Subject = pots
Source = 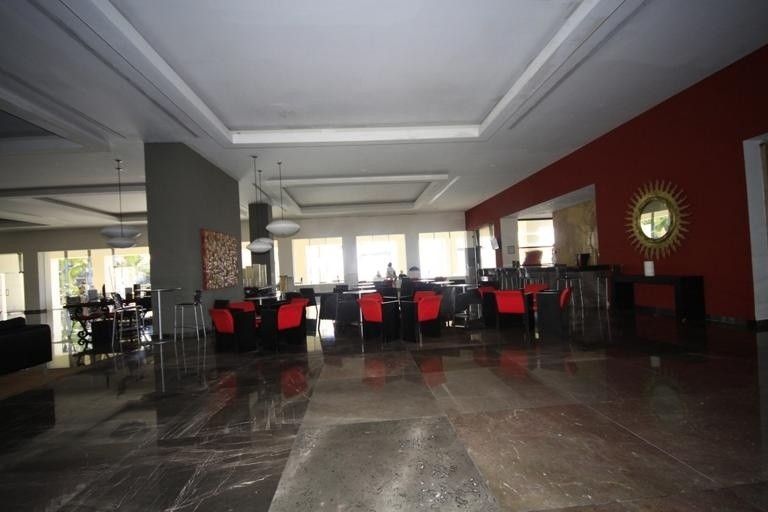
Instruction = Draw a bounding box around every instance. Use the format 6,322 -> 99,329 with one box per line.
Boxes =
576,252 -> 590,267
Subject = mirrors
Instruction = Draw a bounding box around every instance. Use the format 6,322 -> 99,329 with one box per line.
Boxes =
622,179 -> 693,259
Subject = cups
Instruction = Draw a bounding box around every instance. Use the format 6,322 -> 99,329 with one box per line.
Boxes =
88,288 -> 97,301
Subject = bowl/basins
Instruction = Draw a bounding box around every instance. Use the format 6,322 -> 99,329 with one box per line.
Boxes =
67,296 -> 83,306
242,263 -> 267,288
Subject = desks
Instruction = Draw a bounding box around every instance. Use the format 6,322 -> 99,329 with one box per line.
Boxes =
135,287 -> 183,344
612,274 -> 707,340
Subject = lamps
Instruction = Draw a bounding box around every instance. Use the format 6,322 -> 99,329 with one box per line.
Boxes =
254,170 -> 275,244
105,159 -> 136,248
266,161 -> 300,238
99,168 -> 143,240
246,155 -> 272,254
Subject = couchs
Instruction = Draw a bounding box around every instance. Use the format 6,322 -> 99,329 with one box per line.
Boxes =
0,388 -> 55,456
0,316 -> 52,377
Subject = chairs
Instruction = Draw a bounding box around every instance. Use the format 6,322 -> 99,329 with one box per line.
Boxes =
472,331 -> 577,386
319,337 -> 462,391
174,290 -> 206,343
64,284 -> 149,358
204,361 -> 311,435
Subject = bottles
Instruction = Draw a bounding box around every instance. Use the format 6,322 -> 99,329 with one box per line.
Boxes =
336,274 -> 340,283
300,276 -> 303,284
125,284 -> 140,298
511,257 -> 520,268
